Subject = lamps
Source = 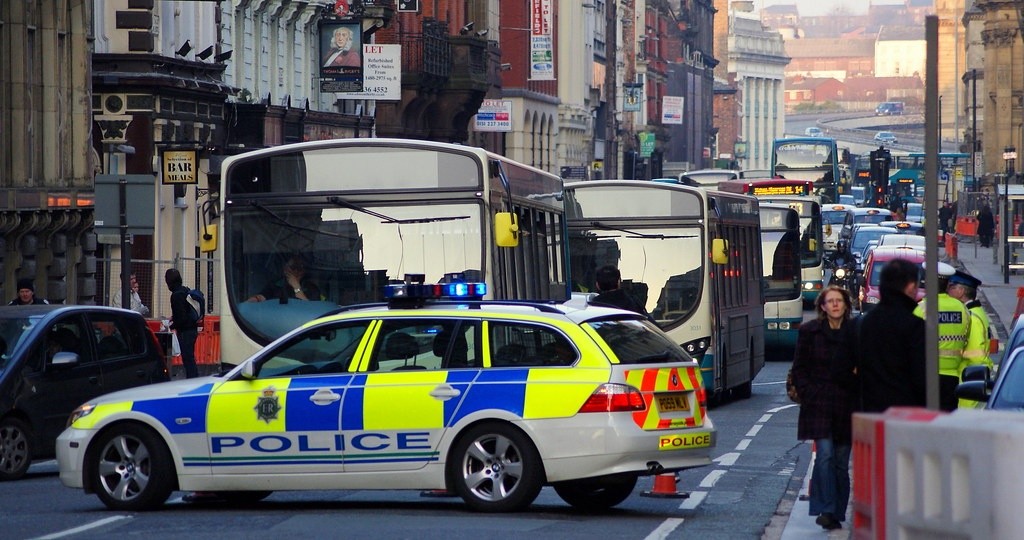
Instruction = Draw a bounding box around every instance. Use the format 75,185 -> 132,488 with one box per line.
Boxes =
214,50 -> 233,62
109,144 -> 135,154
175,40 -> 191,57
475,29 -> 489,38
195,45 -> 213,60
461,22 -> 475,35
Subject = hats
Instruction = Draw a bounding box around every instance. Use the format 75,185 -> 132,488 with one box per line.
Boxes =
920,260 -> 957,283
17,278 -> 34,292
948,270 -> 982,288
165,269 -> 180,285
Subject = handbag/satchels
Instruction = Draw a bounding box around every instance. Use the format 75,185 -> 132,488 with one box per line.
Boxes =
168,327 -> 181,357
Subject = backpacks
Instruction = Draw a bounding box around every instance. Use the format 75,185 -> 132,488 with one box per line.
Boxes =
172,287 -> 205,325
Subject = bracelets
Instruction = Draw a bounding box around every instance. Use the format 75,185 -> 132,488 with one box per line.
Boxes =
294,288 -> 302,294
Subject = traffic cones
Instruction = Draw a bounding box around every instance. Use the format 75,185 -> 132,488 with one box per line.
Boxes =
640,473 -> 690,499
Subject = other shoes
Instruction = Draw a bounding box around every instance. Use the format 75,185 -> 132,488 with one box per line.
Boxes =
816,513 -> 841,529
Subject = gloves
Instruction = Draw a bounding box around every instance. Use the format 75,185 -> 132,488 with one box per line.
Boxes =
132,303 -> 149,314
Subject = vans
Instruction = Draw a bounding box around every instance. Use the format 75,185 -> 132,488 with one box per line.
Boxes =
875,102 -> 904,117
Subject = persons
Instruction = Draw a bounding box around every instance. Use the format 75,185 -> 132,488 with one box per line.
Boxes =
841,258 -> 929,412
976,205 -> 994,248
592,263 -> 640,314
792,287 -> 860,529
7,279 -> 48,305
165,268 -> 199,379
891,190 -> 908,221
946,270 -> 994,413
244,256 -> 322,302
112,271 -> 149,318
910,260 -> 971,414
939,200 -> 956,243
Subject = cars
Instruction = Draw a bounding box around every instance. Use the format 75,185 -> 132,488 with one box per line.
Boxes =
0,304 -> 173,484
54,275 -> 717,513
956,314 -> 1024,412
875,131 -> 899,144
805,127 -> 823,138
819,185 -> 940,314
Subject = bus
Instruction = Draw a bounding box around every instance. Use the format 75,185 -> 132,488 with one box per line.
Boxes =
561,177 -> 765,412
837,147 -> 979,207
198,137 -> 571,378
651,167 -> 833,356
768,136 -> 840,205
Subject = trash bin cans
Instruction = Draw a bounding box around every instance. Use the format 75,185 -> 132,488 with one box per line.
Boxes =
170,314 -> 220,374
992,238 -> 1000,264
956,216 -> 979,236
154,332 -> 174,371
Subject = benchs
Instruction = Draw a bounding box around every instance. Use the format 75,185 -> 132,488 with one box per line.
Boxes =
496,342 -> 572,365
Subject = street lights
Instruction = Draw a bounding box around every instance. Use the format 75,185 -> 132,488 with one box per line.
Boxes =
1002,145 -> 1016,284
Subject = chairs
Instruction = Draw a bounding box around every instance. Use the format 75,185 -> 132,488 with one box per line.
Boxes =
384,328 -> 469,370
44,326 -> 83,363
310,276 -> 331,302
